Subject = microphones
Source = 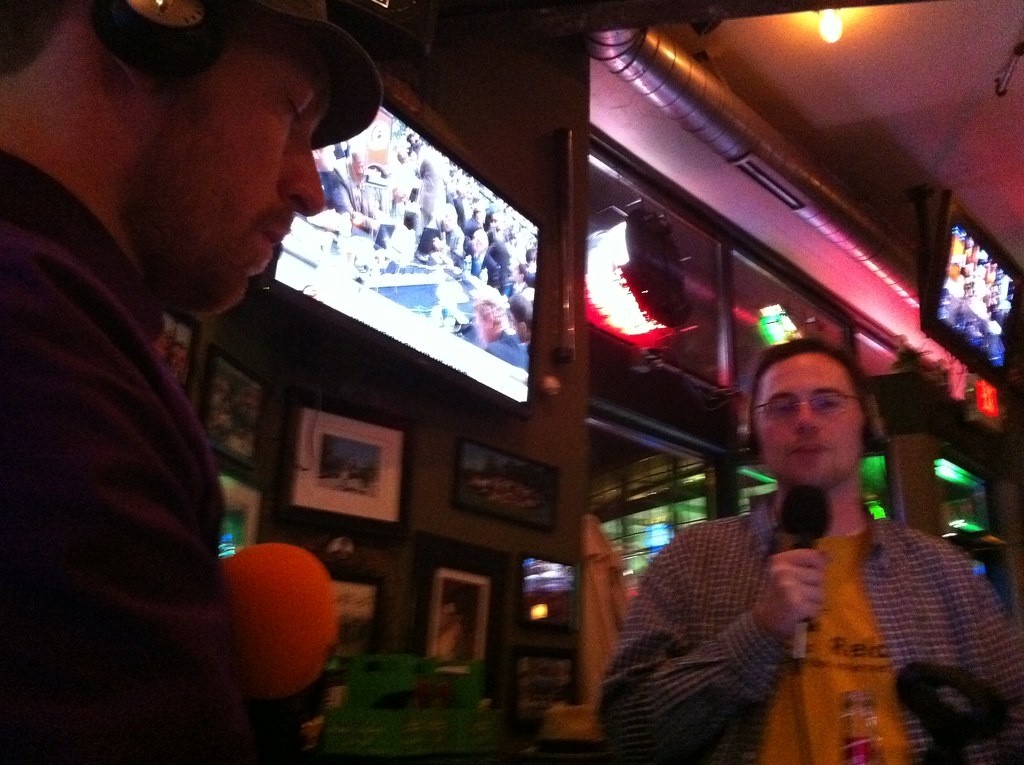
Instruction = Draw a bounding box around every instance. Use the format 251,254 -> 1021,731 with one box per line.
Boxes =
224,543 -> 338,765
782,484 -> 832,661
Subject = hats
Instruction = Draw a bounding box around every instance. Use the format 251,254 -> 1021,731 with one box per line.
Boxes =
206,0 -> 383,147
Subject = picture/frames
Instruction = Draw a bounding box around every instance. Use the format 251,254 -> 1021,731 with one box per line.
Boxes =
152,302 -> 205,399
450,437 -> 561,531
508,643 -> 578,731
269,387 -> 416,541
515,552 -> 581,632
403,530 -> 510,699
199,344 -> 273,470
216,462 -> 269,560
323,562 -> 385,670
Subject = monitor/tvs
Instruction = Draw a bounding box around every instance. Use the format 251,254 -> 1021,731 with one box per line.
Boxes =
921,186 -> 1024,387
255,93 -> 548,420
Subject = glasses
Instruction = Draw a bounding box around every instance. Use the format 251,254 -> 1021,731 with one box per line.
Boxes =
752,392 -> 863,422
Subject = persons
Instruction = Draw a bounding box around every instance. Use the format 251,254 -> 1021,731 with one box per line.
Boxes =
961,233 -> 1006,322
595,338 -> 1024,765
312,122 -> 538,368
0,0 -> 378,765
